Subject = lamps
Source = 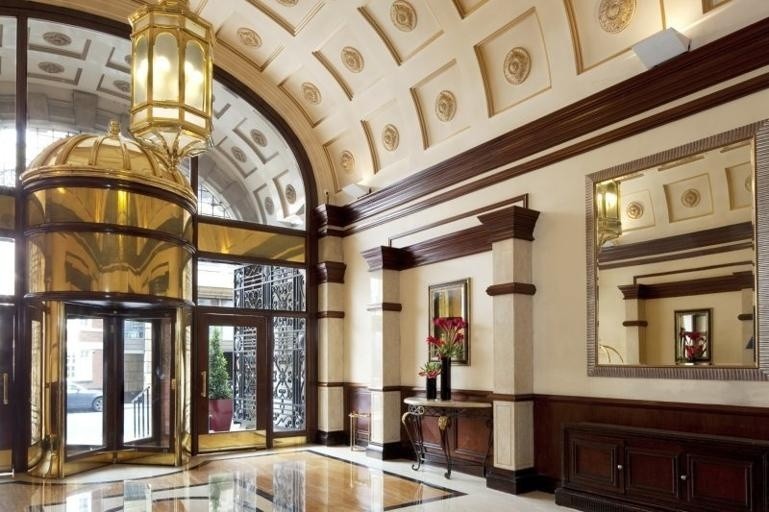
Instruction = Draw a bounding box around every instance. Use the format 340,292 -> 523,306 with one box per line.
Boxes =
595,178 -> 623,256
126,2 -> 219,175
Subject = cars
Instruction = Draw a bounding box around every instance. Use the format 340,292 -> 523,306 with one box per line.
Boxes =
66,380 -> 103,413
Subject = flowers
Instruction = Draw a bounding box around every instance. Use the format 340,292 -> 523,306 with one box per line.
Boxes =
679,325 -> 707,346
418,361 -> 442,378
426,315 -> 469,361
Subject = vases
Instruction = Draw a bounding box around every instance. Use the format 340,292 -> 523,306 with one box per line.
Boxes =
440,355 -> 452,400
685,346 -> 702,362
426,376 -> 436,400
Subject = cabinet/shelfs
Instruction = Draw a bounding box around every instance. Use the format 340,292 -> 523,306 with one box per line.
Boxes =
555,419 -> 769,511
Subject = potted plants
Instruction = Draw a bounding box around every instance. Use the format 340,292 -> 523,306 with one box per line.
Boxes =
208,325 -> 236,431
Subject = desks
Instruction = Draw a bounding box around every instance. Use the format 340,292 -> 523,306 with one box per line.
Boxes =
400,395 -> 493,479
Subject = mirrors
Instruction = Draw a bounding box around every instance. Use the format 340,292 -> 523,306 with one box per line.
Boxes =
673,307 -> 713,364
584,117 -> 768,384
427,275 -> 474,367
